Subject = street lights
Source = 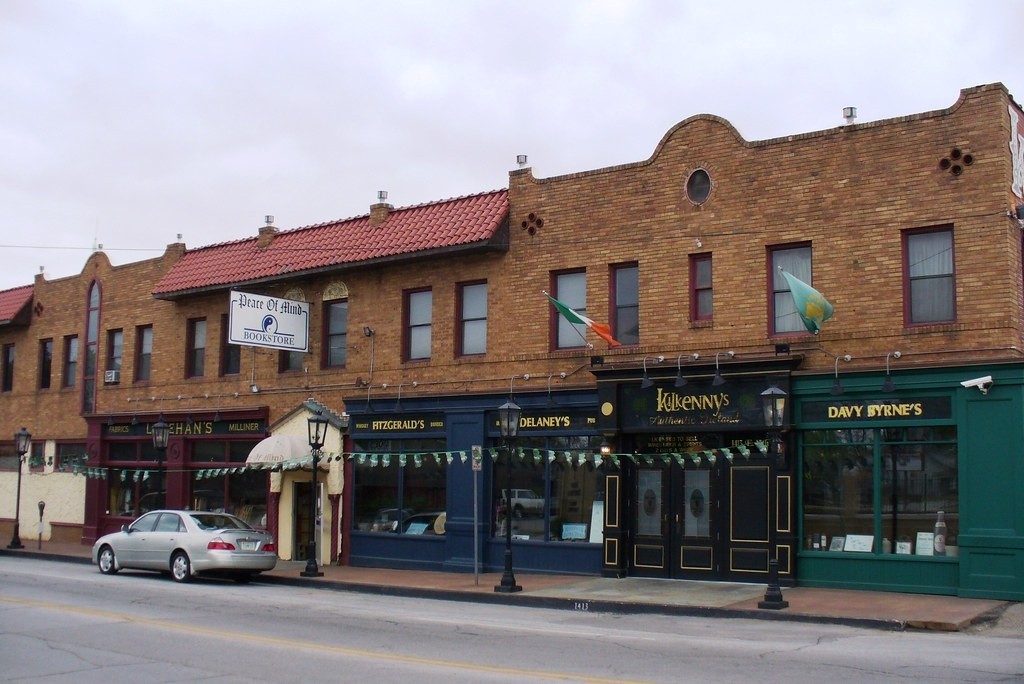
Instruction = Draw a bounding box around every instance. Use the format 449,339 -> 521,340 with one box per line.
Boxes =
6,427 -> 31,549
758,385 -> 789,610
493,398 -> 523,593
300,411 -> 329,577
882,428 -> 904,553
151,412 -> 171,508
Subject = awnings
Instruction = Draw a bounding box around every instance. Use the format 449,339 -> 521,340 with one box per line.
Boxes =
246,434 -> 329,472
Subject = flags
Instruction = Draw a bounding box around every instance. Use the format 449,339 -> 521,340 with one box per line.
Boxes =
782,271 -> 833,332
547,294 -> 621,346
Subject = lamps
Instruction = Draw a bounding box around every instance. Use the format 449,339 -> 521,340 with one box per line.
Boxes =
674,353 -> 700,388
364,384 -> 387,414
642,356 -> 663,388
712,352 -> 733,387
544,372 -> 566,409
394,381 -> 418,412
106,397 -> 226,424
830,355 -> 851,394
507,373 -> 530,404
881,351 -> 902,393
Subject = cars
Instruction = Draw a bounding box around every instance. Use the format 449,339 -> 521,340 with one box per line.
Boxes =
373,509 -> 414,530
91,509 -> 278,583
402,512 -> 446,535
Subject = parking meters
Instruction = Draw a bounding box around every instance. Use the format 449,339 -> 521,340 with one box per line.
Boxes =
37,500 -> 45,549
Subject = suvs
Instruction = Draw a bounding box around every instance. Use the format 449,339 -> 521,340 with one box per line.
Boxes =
500,489 -> 544,519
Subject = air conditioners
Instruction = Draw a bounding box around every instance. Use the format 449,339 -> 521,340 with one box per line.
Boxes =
105,370 -> 119,383
